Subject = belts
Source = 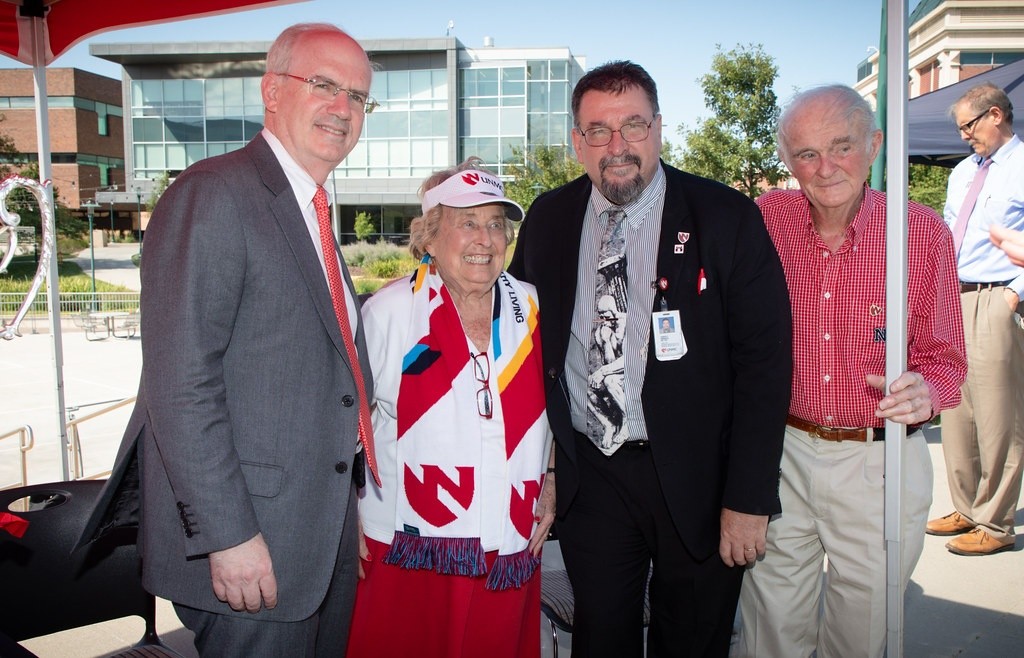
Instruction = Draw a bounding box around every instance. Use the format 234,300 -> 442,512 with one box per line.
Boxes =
957,282 -> 1012,293
788,416 -> 921,443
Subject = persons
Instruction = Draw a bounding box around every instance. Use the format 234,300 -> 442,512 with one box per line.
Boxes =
947,81 -> 1024,556
989,224 -> 1024,268
360,156 -> 557,658
70,22 -> 382,658
506,60 -> 792,658
736,85 -> 966,658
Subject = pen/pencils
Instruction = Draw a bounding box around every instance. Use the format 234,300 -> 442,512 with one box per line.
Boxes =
697,268 -> 707,295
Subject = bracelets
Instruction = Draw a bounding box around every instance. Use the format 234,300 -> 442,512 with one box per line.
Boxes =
547,468 -> 555,473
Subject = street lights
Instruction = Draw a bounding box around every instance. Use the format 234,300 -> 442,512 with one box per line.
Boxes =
110,198 -> 115,243
80,197 -> 102,311
132,186 -> 145,255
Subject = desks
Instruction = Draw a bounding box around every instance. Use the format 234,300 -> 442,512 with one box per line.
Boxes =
88,312 -> 129,338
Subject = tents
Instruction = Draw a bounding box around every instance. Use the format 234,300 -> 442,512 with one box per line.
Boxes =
0,0 -> 309,483
907,57 -> 1024,167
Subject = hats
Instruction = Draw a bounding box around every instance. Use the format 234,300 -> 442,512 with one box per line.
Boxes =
422,169 -> 525,224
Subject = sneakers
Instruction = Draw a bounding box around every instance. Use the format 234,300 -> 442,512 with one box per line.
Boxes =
926,510 -> 978,536
944,528 -> 1015,556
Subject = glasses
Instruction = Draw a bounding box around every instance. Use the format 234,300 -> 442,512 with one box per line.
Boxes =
955,108 -> 990,136
273,71 -> 380,117
575,118 -> 659,146
470,349 -> 498,420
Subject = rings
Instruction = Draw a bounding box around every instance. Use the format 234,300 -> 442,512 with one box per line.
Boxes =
744,547 -> 756,551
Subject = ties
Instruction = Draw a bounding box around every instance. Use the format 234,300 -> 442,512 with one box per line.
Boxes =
586,208 -> 633,457
311,183 -> 384,491
951,159 -> 994,266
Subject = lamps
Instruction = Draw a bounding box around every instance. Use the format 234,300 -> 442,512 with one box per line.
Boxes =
867,47 -> 878,51
446,20 -> 454,36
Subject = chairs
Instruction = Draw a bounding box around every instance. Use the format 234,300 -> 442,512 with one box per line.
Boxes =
537,520 -> 653,658
0,480 -> 185,658
71,308 -> 140,341
